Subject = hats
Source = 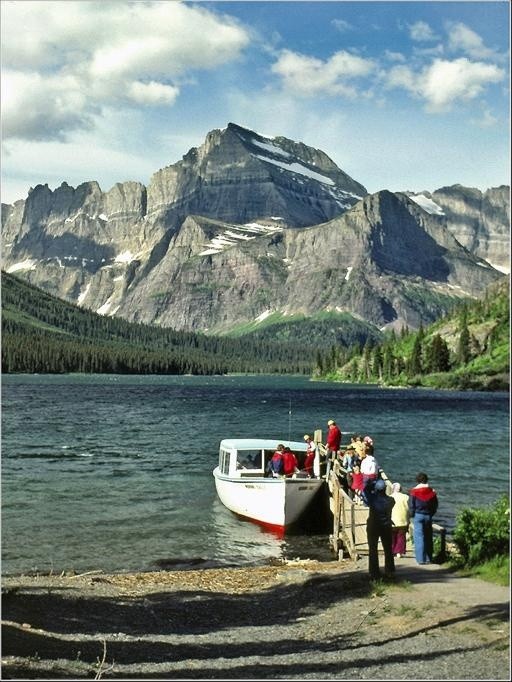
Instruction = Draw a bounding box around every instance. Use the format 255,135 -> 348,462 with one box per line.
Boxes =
346,445 -> 355,450
303,435 -> 310,440
328,420 -> 334,426
375,480 -> 386,491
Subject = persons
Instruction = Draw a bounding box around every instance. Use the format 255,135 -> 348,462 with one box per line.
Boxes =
269,420 -> 410,579
408,471 -> 438,565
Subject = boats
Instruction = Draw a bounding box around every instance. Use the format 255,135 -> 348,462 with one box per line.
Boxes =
212,438 -> 325,541
